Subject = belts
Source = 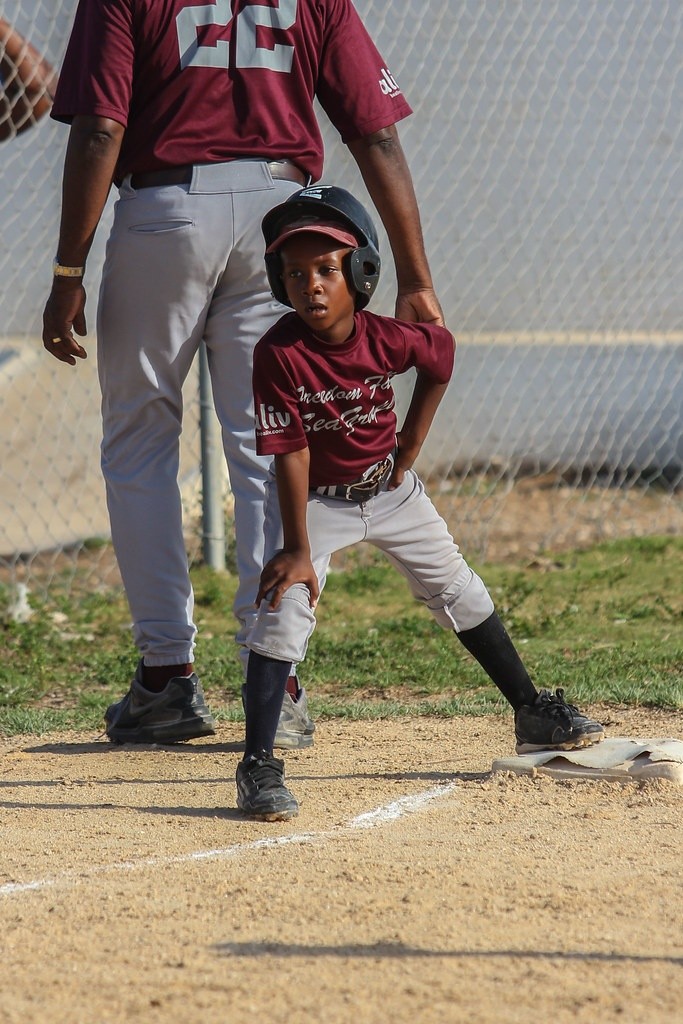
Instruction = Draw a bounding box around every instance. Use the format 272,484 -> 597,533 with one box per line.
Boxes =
310,459 -> 394,501
130,159 -> 309,189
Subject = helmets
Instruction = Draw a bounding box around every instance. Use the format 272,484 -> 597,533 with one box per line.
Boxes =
262,185 -> 381,310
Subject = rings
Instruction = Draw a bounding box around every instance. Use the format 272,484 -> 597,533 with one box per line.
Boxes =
52,336 -> 62,344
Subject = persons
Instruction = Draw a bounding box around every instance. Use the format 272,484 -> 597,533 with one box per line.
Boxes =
0,11 -> 58,152
236,184 -> 601,823
40,0 -> 445,746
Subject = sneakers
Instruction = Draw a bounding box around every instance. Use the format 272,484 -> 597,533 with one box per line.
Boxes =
236,749 -> 299,821
241,675 -> 316,749
98,655 -> 215,744
514,689 -> 605,754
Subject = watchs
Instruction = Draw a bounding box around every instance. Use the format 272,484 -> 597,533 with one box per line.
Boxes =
47,254 -> 88,280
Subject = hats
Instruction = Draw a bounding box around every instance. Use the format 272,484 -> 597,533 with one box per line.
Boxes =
266,209 -> 360,253
